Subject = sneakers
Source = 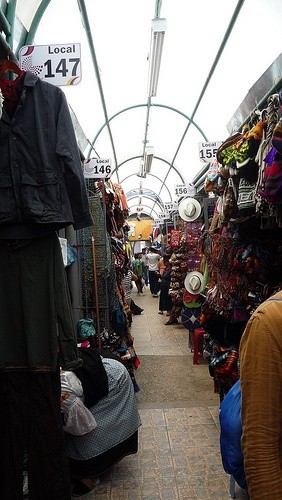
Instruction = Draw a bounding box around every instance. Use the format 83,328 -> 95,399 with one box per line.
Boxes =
166,313 -> 170,316
158,311 -> 163,314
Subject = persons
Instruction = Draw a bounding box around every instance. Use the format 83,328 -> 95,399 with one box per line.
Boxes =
240,291 -> 282,500
121,247 -> 178,325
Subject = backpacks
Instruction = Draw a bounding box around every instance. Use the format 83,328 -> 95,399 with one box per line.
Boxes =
219,378 -> 247,489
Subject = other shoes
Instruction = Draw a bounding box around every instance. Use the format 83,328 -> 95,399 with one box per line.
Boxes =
153,294 -> 158,297
134,308 -> 144,315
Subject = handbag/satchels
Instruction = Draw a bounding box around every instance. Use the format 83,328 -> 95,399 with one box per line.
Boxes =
78,318 -> 96,338
204,90 -> 282,222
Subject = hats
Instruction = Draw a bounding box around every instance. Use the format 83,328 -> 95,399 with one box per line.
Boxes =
181,308 -> 201,331
185,271 -> 205,294
179,198 -> 202,221
183,290 -> 202,307
